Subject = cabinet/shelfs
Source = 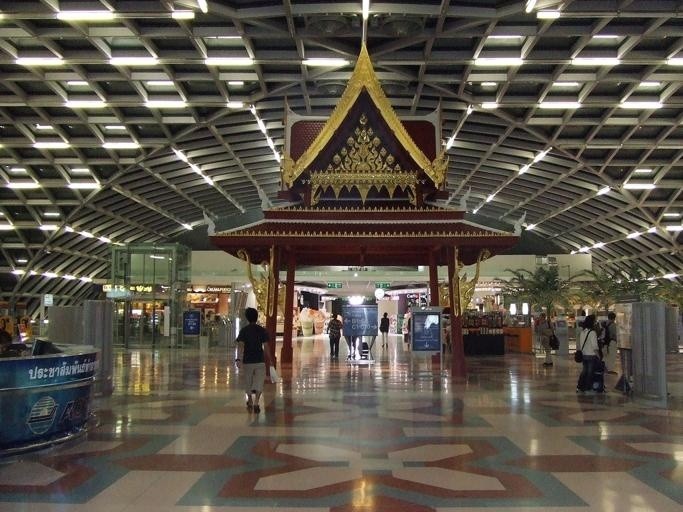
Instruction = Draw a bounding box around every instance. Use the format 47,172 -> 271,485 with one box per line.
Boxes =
406,289 -> 431,308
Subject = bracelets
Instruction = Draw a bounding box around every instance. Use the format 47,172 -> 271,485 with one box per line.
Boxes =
236,359 -> 240,362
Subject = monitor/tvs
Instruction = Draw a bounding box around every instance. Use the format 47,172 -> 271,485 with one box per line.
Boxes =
31,337 -> 51,355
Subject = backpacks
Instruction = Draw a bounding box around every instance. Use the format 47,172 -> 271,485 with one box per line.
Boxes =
597,321 -> 616,354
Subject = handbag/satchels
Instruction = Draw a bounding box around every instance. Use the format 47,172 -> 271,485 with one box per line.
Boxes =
575,350 -> 583,362
269,366 -> 280,384
550,335 -> 560,350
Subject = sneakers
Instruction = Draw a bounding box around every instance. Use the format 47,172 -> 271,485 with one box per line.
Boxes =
608,371 -> 617,374
246,401 -> 253,408
544,362 -> 553,367
382,344 -> 388,348
254,408 -> 260,413
615,385 -> 633,392
576,388 -> 597,394
331,356 -> 338,359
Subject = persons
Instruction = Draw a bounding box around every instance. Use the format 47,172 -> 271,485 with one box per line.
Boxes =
407,318 -> 411,348
22,319 -> 28,328
379,312 -> 389,348
576,312 -> 618,395
0,319 -> 6,332
236,307 -> 274,414
327,313 -> 343,357
0,334 -> 9,357
537,313 -> 554,367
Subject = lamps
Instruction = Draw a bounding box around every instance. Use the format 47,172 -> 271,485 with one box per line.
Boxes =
374,288 -> 391,300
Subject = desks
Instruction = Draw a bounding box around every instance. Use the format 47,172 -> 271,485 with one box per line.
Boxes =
503,327 -> 532,353
463,335 -> 505,355
